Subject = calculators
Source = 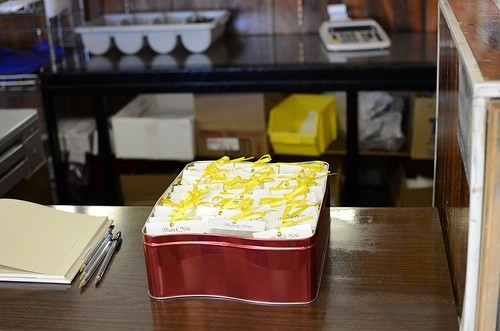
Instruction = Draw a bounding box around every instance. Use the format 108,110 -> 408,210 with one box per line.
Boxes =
321,17 -> 391,52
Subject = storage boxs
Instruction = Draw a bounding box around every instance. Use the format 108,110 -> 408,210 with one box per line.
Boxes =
119,172 -> 177,206
198,93 -> 279,157
141,161 -> 330,305
268,94 -> 337,156
408,93 -> 436,159
388,164 -> 433,207
111,92 -> 197,161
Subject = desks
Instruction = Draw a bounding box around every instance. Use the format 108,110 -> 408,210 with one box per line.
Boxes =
0,205 -> 460,331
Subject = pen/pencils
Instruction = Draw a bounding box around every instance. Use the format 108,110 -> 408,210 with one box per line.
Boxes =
93,231 -> 121,284
79,225 -> 115,271
79,239 -> 112,288
80,232 -> 112,282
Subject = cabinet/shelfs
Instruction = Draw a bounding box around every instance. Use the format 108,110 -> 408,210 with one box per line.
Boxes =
0,0 -> 90,205
38,31 -> 439,208
